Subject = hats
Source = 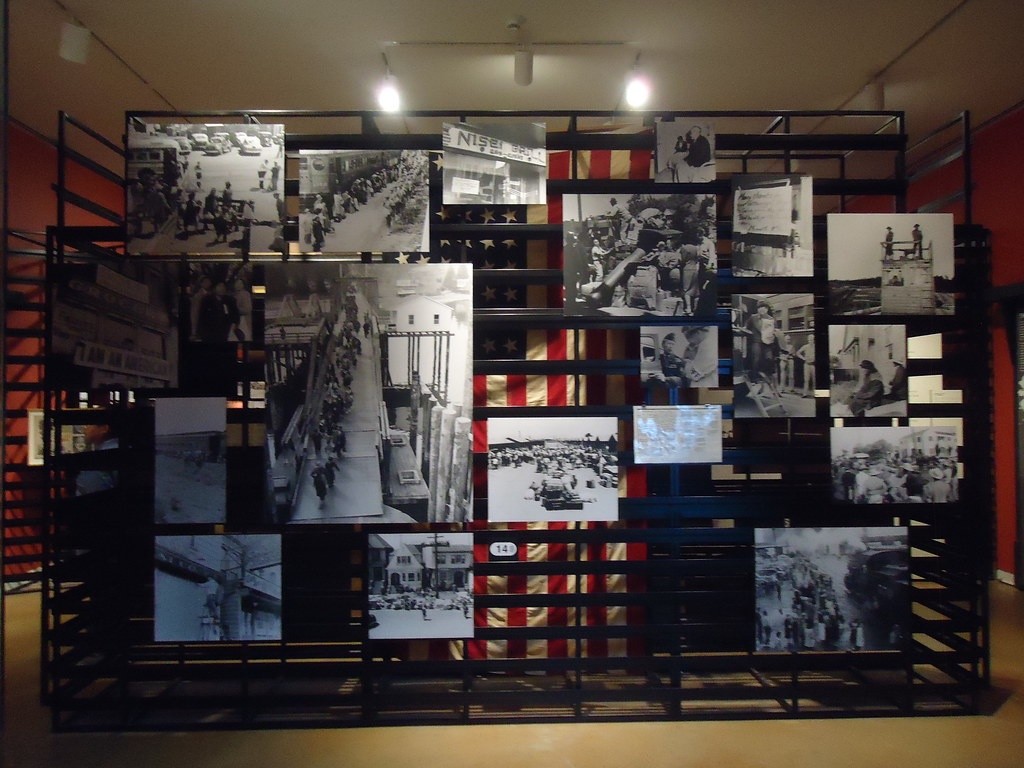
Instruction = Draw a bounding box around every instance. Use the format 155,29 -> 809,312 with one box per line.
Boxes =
859,465 -> 868,469
899,463 -> 914,472
900,475 -> 929,488
913,224 -> 920,227
859,359 -> 878,372
929,468 -> 943,479
759,300 -> 771,308
886,227 -> 892,229
609,198 -> 617,203
867,468 -> 882,475
888,355 -> 904,365
316,194 -> 322,199
912,465 -> 921,472
662,332 -> 676,344
205,273 -> 227,291
944,463 -> 957,475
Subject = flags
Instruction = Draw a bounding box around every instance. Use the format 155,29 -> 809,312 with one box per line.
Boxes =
382,146 -> 655,677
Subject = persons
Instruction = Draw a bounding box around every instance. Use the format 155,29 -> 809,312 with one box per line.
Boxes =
190,274 -> 253,343
68,402 -> 121,556
660,326 -> 718,388
303,154 -> 429,253
197,576 -> 220,619
847,358 -> 907,417
674,125 -> 710,182
488,445 -> 607,496
308,286 -> 371,501
744,303 -> 816,398
831,448 -> 959,503
756,573 -> 863,650
181,445 -> 205,473
378,582 -> 473,620
882,224 -> 925,260
564,196 -> 717,316
139,159 -> 284,243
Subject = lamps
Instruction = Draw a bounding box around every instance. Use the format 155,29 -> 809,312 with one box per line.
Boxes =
514,49 -> 534,87
865,83 -> 885,111
56,21 -> 91,67
